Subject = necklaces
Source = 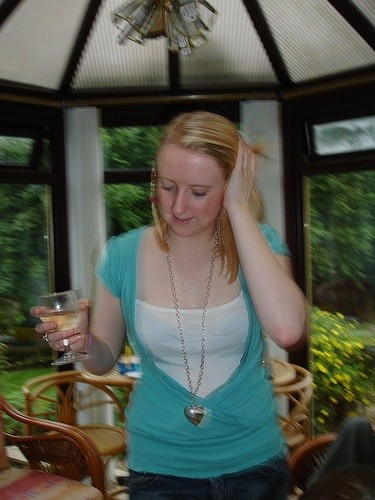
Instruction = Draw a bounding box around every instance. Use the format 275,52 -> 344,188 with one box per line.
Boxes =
163,218 -> 220,426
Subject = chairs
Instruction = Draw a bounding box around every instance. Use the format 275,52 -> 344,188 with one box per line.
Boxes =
0,365 -> 337,500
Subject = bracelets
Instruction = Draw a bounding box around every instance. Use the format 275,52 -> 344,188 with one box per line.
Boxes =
84,331 -> 93,354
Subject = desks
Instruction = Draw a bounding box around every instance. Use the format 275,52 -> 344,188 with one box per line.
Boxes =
72,358 -> 297,388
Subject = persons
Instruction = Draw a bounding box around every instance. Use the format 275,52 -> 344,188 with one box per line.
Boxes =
29,110 -> 307,500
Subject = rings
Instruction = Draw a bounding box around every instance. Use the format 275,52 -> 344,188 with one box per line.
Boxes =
41,334 -> 49,342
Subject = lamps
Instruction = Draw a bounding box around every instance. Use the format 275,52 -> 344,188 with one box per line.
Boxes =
111,0 -> 216,56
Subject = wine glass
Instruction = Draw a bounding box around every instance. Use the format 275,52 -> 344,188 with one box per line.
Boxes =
37,290 -> 88,365
